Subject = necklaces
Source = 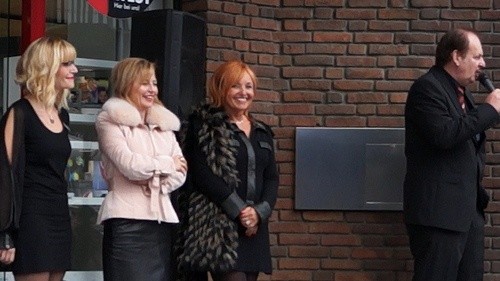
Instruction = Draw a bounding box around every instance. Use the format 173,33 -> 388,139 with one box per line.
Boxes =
42,99 -> 57,124
228,115 -> 246,125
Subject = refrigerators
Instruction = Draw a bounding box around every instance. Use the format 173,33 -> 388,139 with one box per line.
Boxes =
0,55 -> 120,281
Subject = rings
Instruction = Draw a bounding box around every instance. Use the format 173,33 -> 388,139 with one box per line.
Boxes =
246,220 -> 252,225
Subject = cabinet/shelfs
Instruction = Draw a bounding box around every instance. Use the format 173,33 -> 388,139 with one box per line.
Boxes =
4,58 -> 120,207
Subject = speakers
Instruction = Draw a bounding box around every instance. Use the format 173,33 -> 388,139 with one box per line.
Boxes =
130,9 -> 206,149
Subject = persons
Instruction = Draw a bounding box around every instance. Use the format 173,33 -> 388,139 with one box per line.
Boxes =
403,26 -> 500,281
95,57 -> 187,281
0,36 -> 81,281
172,61 -> 279,281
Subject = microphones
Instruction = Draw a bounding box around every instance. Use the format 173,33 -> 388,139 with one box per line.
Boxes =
478,71 -> 495,92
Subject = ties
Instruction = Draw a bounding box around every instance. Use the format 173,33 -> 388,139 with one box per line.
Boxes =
456,86 -> 468,116
